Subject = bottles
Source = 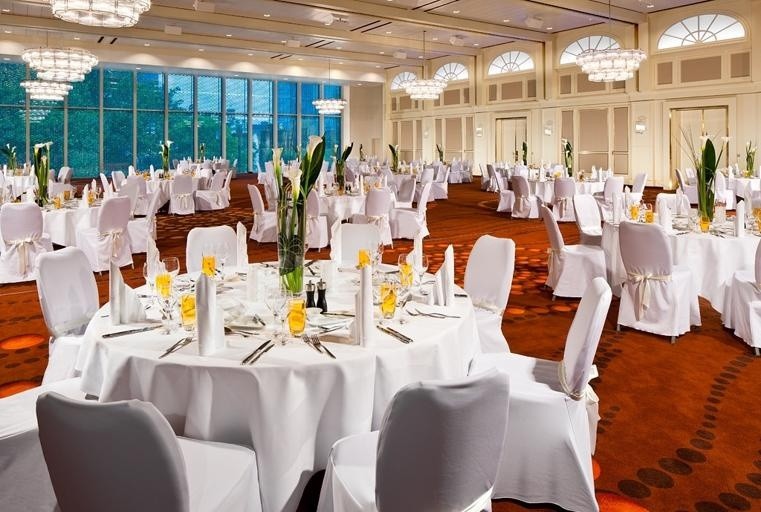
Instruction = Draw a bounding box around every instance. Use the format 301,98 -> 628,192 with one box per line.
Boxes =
304,280 -> 315,307
316,279 -> 327,313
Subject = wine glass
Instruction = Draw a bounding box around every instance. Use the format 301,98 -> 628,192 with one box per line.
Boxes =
273,289 -> 293,346
211,243 -> 228,289
142,261 -> 162,308
94,184 -> 102,201
412,254 -> 428,289
161,257 -> 179,299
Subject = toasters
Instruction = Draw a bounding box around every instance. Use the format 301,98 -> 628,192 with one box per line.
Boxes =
398,254 -> 412,292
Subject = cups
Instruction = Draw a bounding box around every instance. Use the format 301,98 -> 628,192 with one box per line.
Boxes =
699,215 -> 709,233
399,261 -> 413,290
201,255 -> 216,280
644,208 -> 653,222
378,279 -> 397,319
154,272 -> 172,314
357,248 -> 371,268
287,298 -> 306,337
180,292 -> 196,333
630,204 -> 640,221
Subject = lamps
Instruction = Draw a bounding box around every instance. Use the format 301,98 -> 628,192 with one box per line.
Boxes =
403,30 -> 447,100
21,31 -> 98,82
17,78 -> 73,104
44,0 -> 151,29
311,56 -> 347,116
17,100 -> 57,124
575,0 -> 648,83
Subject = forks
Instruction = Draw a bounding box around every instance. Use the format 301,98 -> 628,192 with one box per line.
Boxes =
302,334 -> 322,354
404,309 -> 447,317
414,308 -> 460,318
310,335 -> 336,359
159,337 -> 192,359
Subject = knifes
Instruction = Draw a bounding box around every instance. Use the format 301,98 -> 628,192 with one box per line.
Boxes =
375,325 -> 408,344
248,343 -> 274,364
386,326 -> 413,343
102,324 -> 161,339
241,338 -> 270,363
